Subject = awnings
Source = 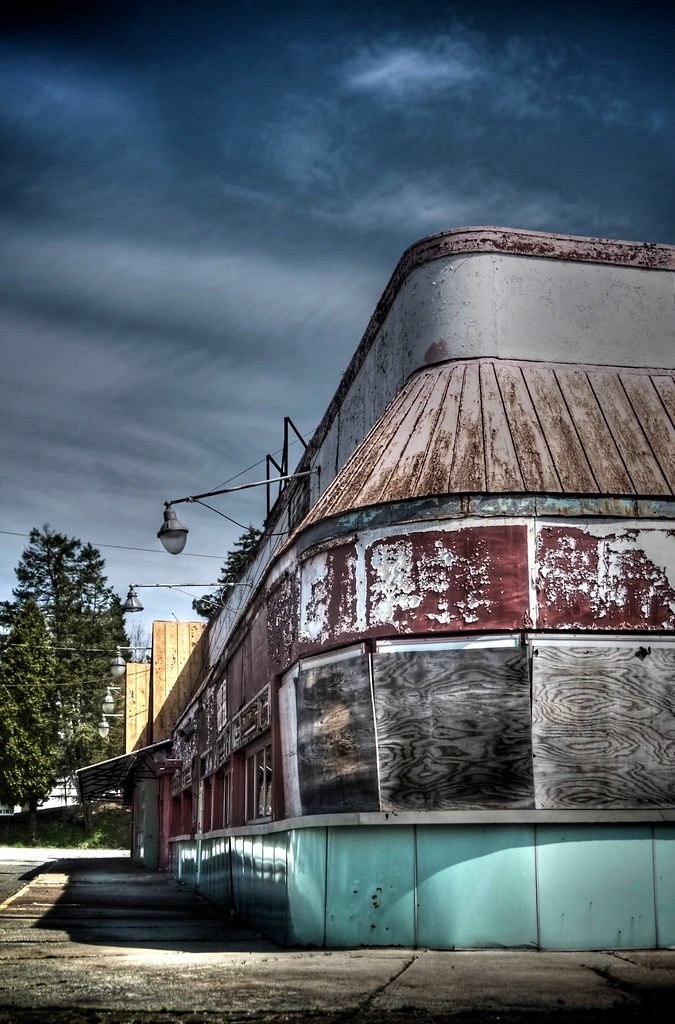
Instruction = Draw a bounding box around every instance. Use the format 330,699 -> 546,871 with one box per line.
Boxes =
73,738 -> 172,802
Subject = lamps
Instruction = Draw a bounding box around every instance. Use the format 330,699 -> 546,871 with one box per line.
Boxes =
122,582 -> 235,612
157,469 -> 318,555
